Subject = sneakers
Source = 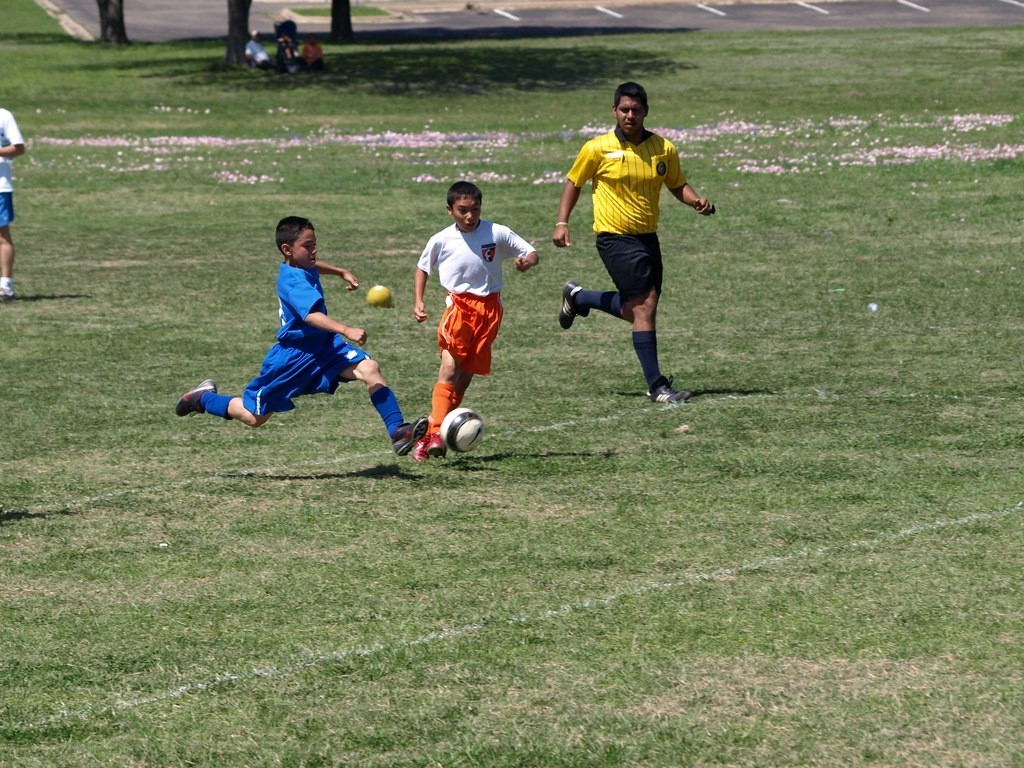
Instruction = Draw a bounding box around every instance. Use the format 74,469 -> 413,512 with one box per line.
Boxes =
426,433 -> 447,458
392,418 -> 428,457
412,428 -> 435,463
647,374 -> 691,404
0,280 -> 13,298
175,379 -> 216,417
559,280 -> 583,330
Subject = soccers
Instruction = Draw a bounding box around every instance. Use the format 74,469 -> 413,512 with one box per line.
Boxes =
439,408 -> 484,453
366,285 -> 392,308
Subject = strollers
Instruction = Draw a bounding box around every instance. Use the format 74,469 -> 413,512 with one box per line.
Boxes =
272,19 -> 306,74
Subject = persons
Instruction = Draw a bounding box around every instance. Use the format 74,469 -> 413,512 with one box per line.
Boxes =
176,215 -> 429,456
245,21 -> 325,73
411,180 -> 539,462
552,80 -> 715,405
0,108 -> 24,296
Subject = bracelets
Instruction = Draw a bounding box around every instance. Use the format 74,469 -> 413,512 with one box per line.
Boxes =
555,222 -> 568,226
